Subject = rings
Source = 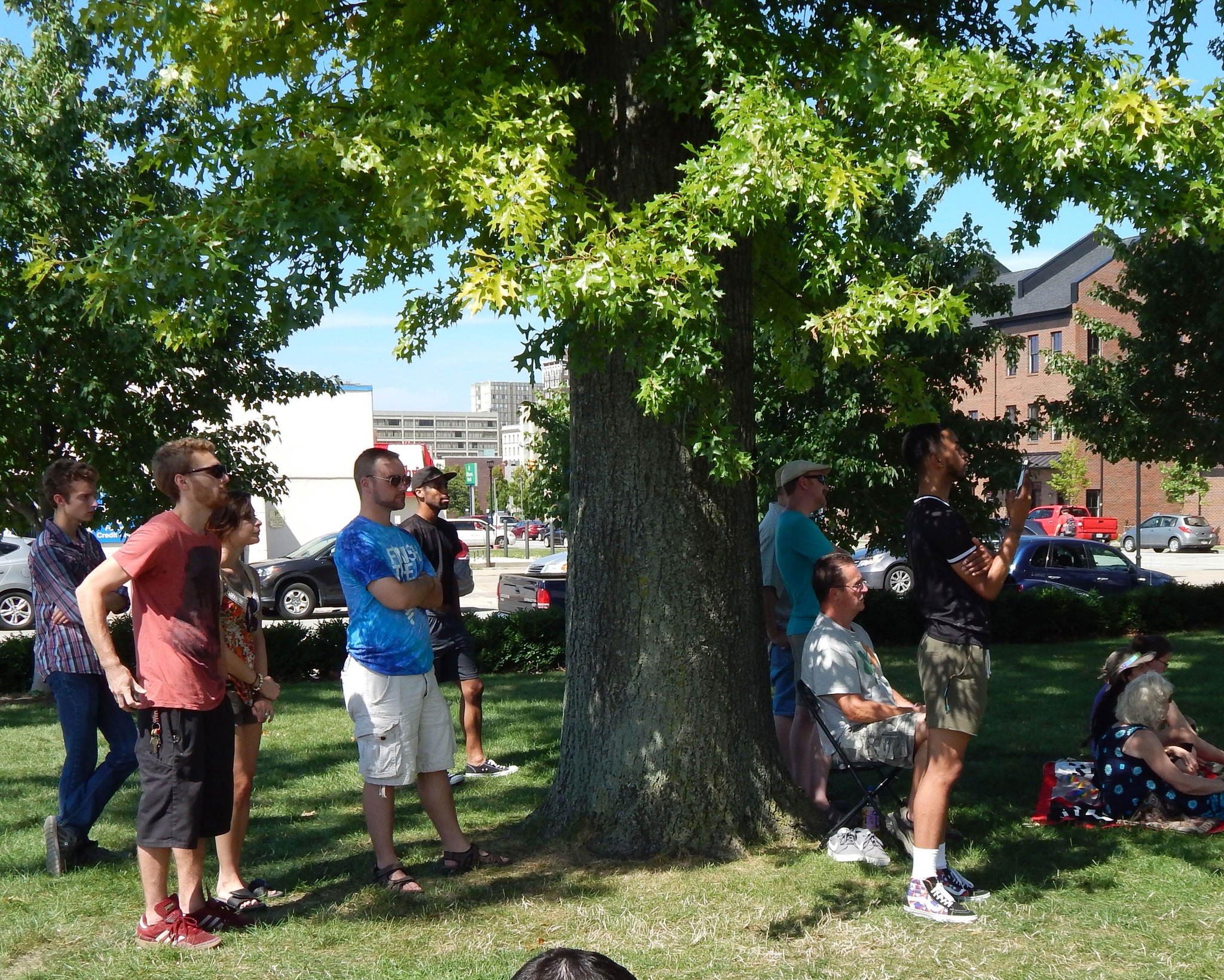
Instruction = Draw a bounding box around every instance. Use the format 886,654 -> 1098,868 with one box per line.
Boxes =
265,712 -> 269,716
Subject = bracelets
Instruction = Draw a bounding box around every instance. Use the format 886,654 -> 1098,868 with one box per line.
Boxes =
251,673 -> 264,691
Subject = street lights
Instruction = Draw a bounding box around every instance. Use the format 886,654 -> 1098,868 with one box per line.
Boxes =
516,478 -> 523,520
486,459 -> 495,525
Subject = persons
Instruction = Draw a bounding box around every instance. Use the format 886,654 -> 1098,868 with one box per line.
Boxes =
398,466 -> 518,786
761,423 -> 1224,921
511,947 -> 638,980
200,489 -> 285,914
75,438 -> 254,949
29,458 -> 139,880
335,448 -> 513,900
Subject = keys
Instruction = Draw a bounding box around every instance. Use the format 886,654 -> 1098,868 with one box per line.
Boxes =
150,729 -> 162,753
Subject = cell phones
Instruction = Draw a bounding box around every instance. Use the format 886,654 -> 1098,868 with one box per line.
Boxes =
1017,459 -> 1029,493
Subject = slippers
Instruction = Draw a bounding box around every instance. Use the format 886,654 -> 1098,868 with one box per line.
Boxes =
815,804 -> 841,821
217,888 -> 266,914
247,877 -> 285,898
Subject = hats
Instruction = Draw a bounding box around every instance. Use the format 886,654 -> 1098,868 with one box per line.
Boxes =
777,464 -> 787,489
1097,647 -> 1157,683
412,466 -> 457,495
782,460 -> 831,487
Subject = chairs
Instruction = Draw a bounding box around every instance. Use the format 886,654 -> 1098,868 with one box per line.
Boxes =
1057,554 -> 1073,566
797,679 -> 907,853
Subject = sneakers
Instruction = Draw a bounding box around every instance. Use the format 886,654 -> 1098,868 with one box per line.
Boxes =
853,827 -> 890,866
75,842 -> 119,863
936,865 -> 990,901
886,808 -> 914,857
903,876 -> 977,923
827,828 -> 866,862
446,771 -> 465,784
465,758 -> 519,778
44,815 -> 82,877
136,894 -> 221,952
185,884 -> 258,931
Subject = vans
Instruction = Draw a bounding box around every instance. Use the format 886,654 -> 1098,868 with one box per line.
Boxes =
457,515 -> 489,524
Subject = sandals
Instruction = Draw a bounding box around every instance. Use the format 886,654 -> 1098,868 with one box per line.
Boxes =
441,842 -> 512,875
372,863 -> 425,894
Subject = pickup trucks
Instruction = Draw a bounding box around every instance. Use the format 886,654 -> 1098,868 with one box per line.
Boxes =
497,572 -> 566,618
1027,505 -> 1118,543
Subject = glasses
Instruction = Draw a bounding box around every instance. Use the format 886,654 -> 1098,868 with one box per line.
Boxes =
1153,657 -> 1169,670
366,474 -> 411,488
804,474 -> 826,486
180,464 -> 228,478
833,579 -> 868,592
1167,695 -> 1173,704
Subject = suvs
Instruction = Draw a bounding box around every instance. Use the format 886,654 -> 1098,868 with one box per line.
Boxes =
988,517 -> 1048,538
511,520 -> 546,541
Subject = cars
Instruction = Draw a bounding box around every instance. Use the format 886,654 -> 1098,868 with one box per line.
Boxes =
488,511 -> 518,532
987,534 -> 1178,602
538,521 -> 552,541
527,551 -> 567,573
244,532 -> 348,620
443,518 -> 515,549
1119,514 -> 1217,553
0,533 -> 37,631
848,537 -> 915,599
543,522 -> 568,548
